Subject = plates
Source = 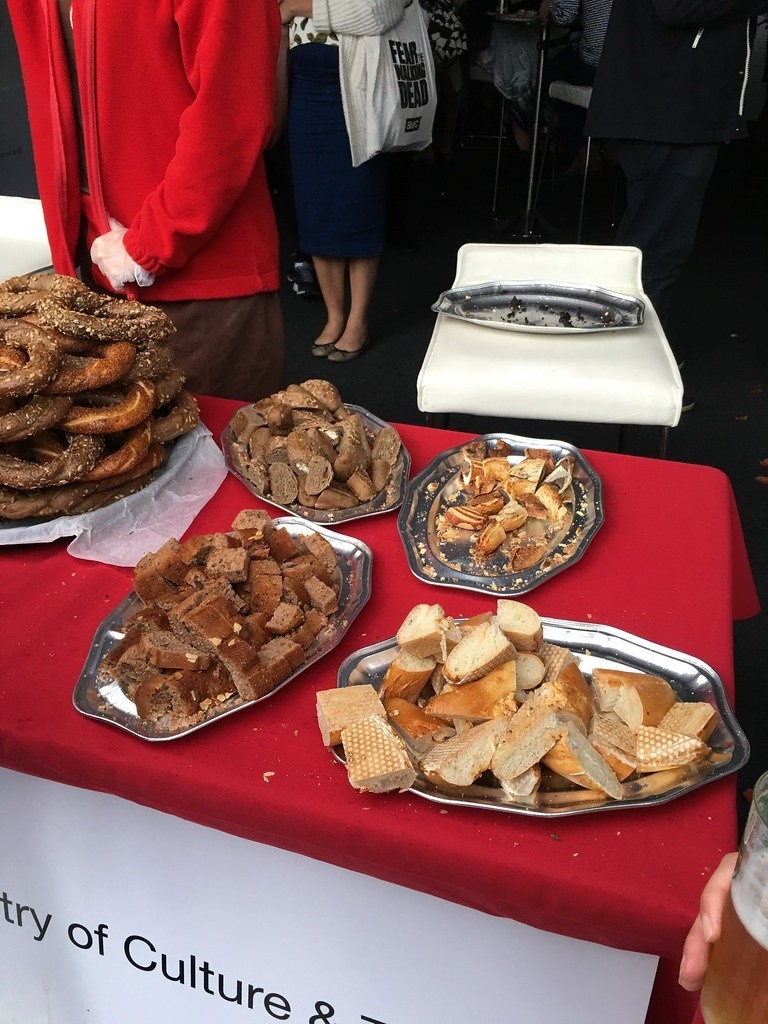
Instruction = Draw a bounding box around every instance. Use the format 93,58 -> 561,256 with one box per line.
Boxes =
218,403 -> 414,529
431,280 -> 647,334
71,516 -> 374,744
328,617 -> 752,818
394,433 -> 603,601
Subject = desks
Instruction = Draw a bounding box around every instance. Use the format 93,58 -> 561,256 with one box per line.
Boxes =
483,10 -> 548,220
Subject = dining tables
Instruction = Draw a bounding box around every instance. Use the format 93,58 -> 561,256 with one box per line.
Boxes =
0,391 -> 763,1023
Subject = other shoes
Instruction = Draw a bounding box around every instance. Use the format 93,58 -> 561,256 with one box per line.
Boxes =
313,331 -> 374,361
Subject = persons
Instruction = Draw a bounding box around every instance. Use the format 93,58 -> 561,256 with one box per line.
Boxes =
2,0 -> 289,412
260,0 -> 766,413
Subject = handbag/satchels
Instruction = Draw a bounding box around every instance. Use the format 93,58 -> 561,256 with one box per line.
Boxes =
336,0 -> 439,168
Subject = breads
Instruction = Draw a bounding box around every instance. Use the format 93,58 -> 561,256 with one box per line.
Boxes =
446,441 -> 579,571
229,378 -> 402,512
0,273 -> 201,527
103,508 -> 339,723
316,596 -> 719,793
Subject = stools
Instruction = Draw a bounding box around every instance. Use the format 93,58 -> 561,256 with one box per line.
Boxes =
418,240 -> 684,460
531,80 -> 593,246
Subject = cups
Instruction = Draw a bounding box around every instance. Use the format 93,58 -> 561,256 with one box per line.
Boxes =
692,769 -> 767,1024
